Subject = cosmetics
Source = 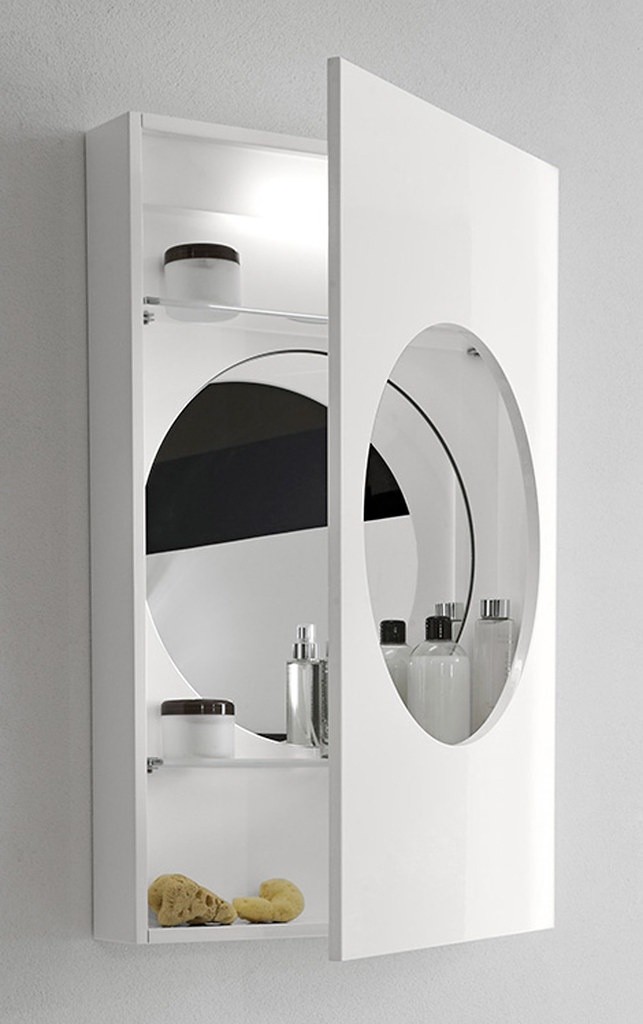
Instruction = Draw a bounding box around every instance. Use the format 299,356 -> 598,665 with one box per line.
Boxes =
469,598 -> 515,733
284,624 -> 324,748
378,619 -> 412,710
316,639 -> 331,760
435,600 -> 466,645
160,699 -> 237,762
405,614 -> 471,747
160,241 -> 243,324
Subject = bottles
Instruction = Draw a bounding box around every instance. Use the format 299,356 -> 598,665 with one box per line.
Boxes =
285,622 -> 320,751
409,597 -> 517,743
379,600 -> 463,708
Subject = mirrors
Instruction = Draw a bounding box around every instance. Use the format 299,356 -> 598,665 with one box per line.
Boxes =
145,381 -> 420,745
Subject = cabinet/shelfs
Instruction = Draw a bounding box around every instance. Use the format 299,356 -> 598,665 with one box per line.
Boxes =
84,56 -> 561,963
